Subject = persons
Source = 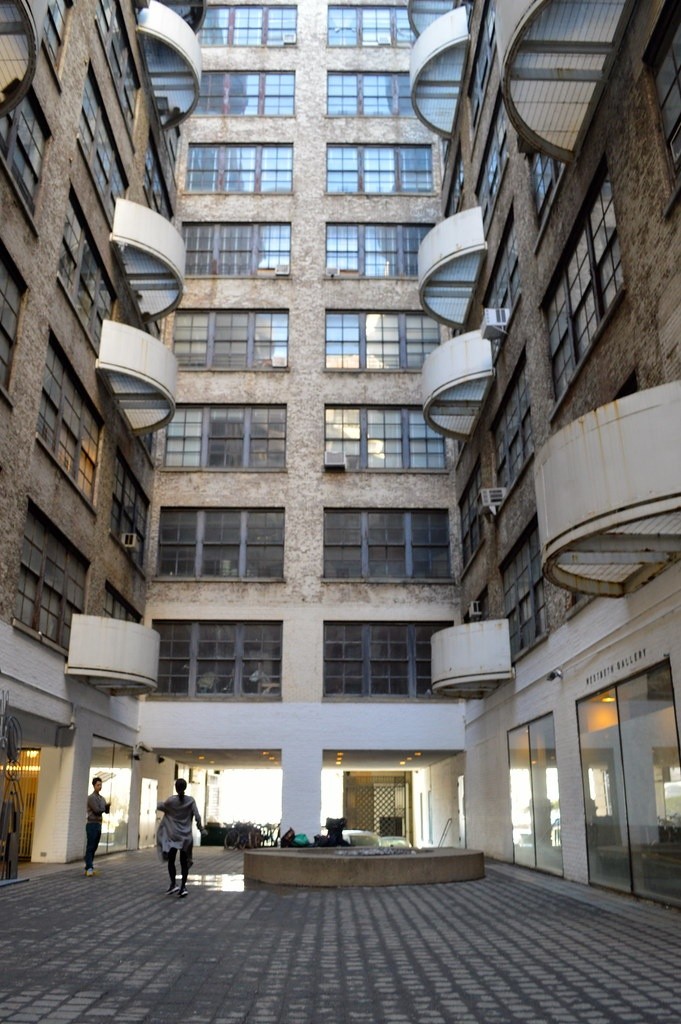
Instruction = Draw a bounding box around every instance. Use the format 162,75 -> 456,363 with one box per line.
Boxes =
155,778 -> 210,897
82,777 -> 112,876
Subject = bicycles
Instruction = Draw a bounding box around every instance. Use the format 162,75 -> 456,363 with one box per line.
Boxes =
224,820 -> 281,850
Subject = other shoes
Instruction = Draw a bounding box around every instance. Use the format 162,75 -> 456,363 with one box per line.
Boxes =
179,888 -> 188,896
165,883 -> 179,895
86,868 -> 93,876
